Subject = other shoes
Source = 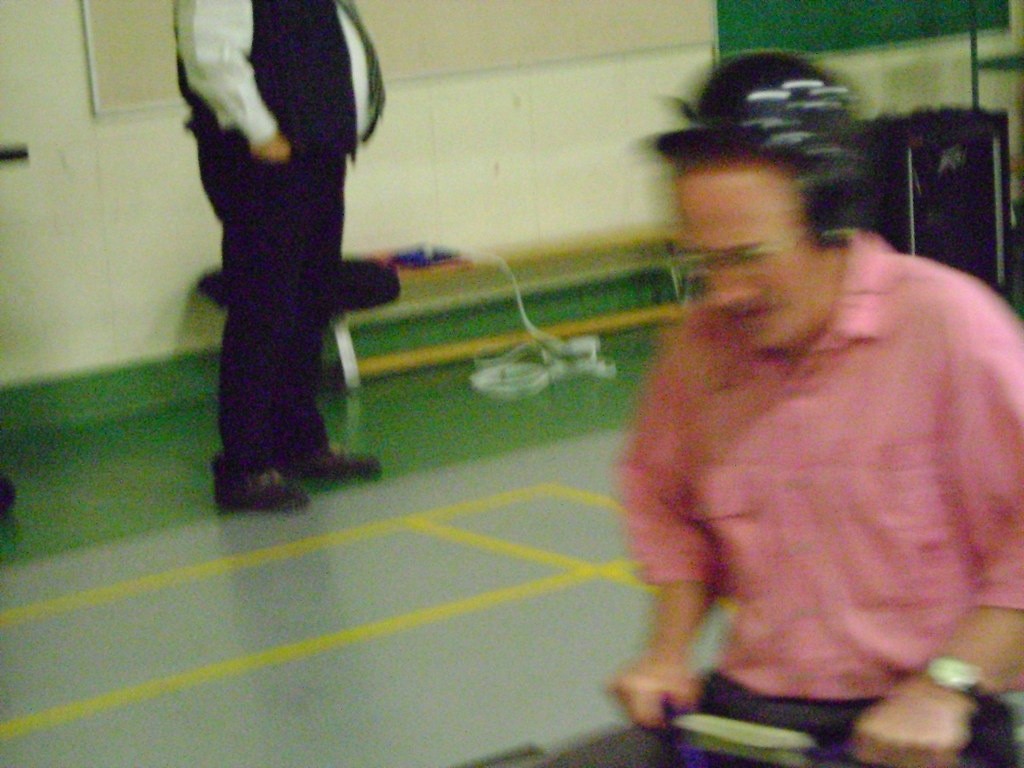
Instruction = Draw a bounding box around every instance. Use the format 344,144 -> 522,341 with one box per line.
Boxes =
286,447 -> 383,484
211,465 -> 293,511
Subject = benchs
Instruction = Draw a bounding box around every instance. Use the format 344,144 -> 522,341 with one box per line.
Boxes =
333,215 -> 701,401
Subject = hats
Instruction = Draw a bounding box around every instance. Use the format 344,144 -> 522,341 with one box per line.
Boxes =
657,55 -> 863,177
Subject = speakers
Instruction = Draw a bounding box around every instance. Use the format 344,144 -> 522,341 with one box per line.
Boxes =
848,106 -> 1010,299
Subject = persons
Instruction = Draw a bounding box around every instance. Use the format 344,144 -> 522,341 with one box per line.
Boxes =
172,0 -> 385,510
531,50 -> 1023,768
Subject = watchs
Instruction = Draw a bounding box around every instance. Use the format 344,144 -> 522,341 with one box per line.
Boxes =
924,656 -> 991,710
